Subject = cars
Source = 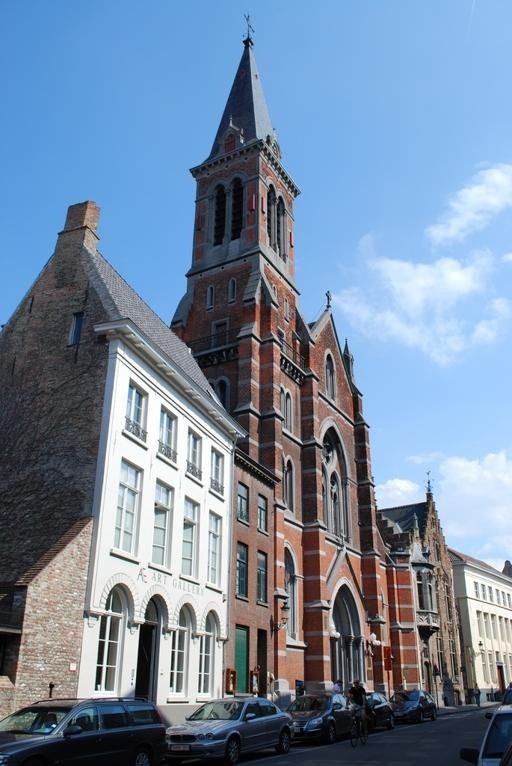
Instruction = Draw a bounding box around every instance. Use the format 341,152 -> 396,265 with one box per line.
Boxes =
165,698 -> 294,765
461,684 -> 512,765
390,689 -> 438,724
285,690 -> 395,748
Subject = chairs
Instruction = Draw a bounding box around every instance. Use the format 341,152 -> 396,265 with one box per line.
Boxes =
45,713 -> 94,731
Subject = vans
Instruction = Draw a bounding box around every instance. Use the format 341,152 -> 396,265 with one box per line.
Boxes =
0,695 -> 167,765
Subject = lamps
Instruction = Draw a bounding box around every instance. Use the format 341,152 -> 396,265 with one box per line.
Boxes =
271,603 -> 290,632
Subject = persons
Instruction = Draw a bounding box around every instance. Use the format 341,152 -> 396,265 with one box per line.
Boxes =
344,680 -> 369,744
473,684 -> 481,707
334,681 -> 343,693
